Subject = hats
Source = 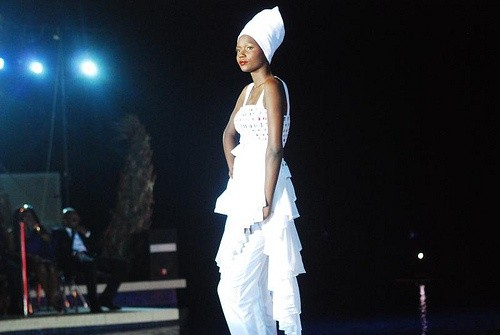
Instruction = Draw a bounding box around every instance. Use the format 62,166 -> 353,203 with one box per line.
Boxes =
237,6 -> 285,64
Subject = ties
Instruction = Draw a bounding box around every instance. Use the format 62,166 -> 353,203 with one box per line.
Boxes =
69,228 -> 75,249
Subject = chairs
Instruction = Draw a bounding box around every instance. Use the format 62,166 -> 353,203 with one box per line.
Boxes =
69,272 -> 111,314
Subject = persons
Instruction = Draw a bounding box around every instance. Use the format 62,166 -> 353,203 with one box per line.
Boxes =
0,200 -> 121,315
213,6 -> 308,335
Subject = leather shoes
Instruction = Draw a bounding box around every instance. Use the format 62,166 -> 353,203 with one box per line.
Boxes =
84,296 -> 121,313
